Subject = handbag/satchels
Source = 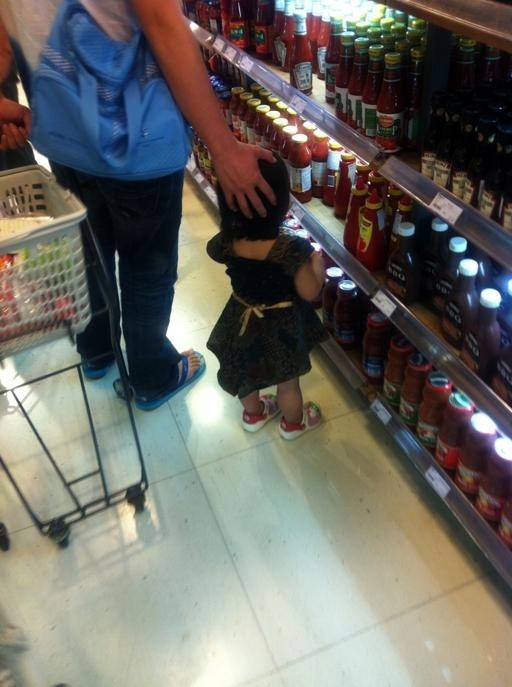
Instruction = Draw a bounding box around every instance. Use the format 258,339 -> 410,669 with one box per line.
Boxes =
28,0 -> 191,181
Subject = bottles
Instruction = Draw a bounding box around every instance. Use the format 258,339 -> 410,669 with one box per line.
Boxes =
423,31 -> 511,236
284,145 -> 510,403
397,354 -> 511,551
183,0 -> 430,185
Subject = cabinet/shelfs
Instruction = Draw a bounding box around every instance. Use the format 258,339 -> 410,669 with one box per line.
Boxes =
184,1 -> 512,582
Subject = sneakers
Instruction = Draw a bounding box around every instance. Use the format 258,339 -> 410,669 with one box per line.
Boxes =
279,401 -> 322,439
243,395 -> 280,432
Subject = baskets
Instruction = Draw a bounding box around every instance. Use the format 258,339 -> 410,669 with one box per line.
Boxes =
0,164 -> 92,359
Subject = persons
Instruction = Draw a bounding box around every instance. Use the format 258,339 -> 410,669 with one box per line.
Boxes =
0,1 -> 278,409
206,145 -> 327,441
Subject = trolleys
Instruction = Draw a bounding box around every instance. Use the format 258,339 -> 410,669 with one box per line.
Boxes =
0,119 -> 151,551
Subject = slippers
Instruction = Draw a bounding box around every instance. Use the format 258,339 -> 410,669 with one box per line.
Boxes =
135,349 -> 205,410
